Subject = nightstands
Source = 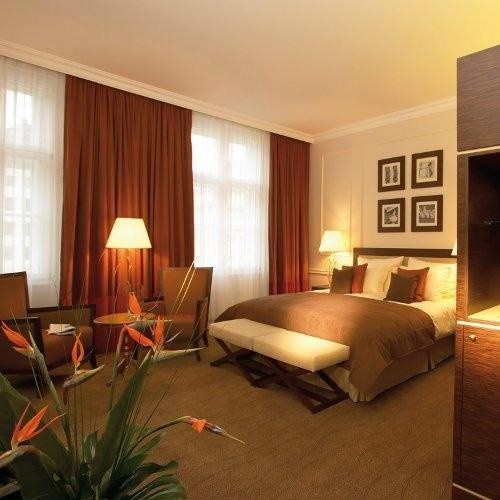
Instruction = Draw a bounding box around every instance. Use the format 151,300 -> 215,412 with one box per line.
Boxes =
312,282 -> 329,290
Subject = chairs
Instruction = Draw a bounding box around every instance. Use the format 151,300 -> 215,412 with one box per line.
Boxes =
0,270 -> 101,400
152,266 -> 214,360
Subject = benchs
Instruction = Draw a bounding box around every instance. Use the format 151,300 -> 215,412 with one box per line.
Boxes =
209,317 -> 352,415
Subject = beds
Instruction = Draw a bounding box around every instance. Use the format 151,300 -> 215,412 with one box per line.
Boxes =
213,245 -> 458,405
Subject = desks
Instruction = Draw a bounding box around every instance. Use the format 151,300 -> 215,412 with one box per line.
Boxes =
93,312 -> 157,386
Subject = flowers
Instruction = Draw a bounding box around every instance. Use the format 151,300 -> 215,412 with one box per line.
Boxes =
0,257 -> 247,500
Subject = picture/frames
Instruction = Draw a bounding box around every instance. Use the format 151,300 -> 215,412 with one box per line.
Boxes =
376,198 -> 406,233
410,195 -> 446,234
377,155 -> 406,193
409,148 -> 445,188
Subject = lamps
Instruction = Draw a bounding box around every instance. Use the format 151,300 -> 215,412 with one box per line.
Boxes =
317,231 -> 346,285
105,217 -> 153,317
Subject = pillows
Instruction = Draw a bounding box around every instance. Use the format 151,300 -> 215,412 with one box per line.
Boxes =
408,255 -> 457,298
383,271 -> 419,303
329,267 -> 353,296
361,267 -> 390,301
357,254 -> 405,288
399,267 -> 431,302
343,264 -> 368,295
402,265 -> 450,302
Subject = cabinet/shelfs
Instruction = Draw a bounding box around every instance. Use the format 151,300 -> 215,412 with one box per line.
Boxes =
448,43 -> 499,500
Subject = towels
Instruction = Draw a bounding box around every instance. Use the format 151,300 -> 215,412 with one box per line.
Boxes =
45,321 -> 78,338
